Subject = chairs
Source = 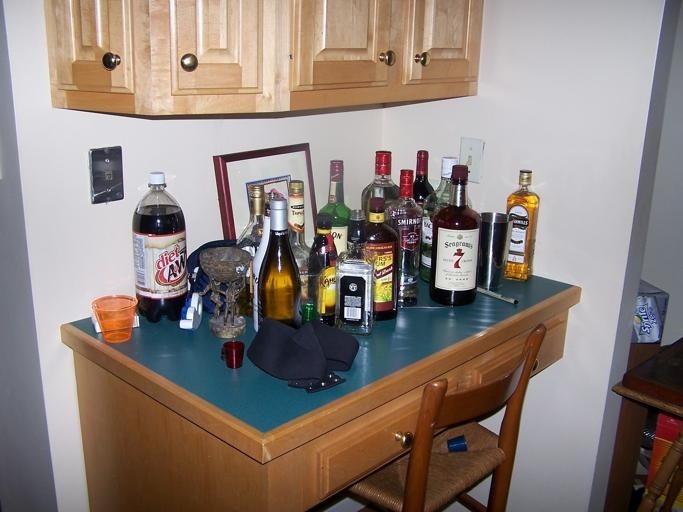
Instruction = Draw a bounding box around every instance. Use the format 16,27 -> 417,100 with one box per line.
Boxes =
343,315 -> 551,512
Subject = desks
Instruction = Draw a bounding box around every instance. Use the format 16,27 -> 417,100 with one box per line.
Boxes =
54,252 -> 585,511
606,335 -> 683,511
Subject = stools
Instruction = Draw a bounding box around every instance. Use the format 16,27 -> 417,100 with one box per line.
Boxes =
647,414 -> 683,505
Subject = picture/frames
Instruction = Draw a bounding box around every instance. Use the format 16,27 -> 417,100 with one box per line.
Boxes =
211,141 -> 320,281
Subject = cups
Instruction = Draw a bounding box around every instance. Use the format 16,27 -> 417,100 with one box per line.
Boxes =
476,212 -> 513,293
91,296 -> 138,344
447,435 -> 466,453
223,342 -> 244,369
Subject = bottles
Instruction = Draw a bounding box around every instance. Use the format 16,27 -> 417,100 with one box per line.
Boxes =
501,169 -> 539,282
132,172 -> 187,322
236,150 -> 480,335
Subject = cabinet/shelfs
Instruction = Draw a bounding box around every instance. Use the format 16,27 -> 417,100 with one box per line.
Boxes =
42,0 -> 277,120
277,0 -> 483,116
602,341 -> 662,511
